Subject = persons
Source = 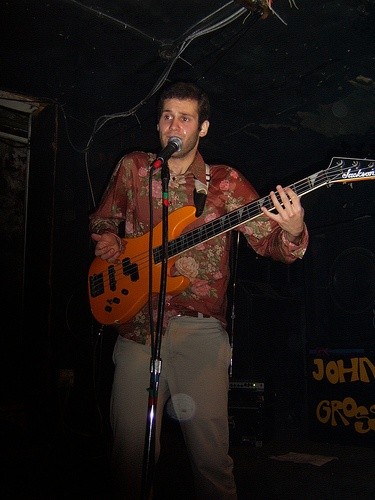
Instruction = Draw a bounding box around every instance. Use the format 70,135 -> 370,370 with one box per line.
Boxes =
90,83 -> 309,500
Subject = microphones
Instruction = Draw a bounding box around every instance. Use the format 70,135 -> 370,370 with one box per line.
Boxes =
151,137 -> 183,171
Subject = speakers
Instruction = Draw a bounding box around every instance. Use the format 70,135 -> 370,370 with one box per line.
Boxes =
298,211 -> 375,352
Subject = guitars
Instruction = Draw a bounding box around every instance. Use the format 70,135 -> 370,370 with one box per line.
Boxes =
87,161 -> 375,324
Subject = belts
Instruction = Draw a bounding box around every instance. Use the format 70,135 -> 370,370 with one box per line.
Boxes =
177,309 -> 210,319
